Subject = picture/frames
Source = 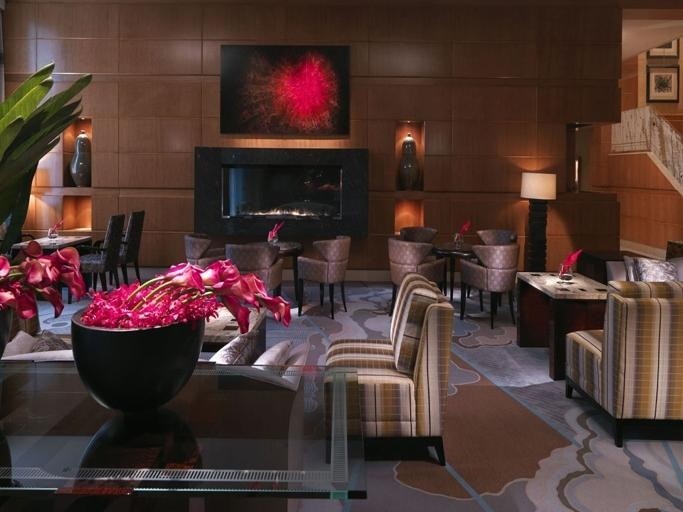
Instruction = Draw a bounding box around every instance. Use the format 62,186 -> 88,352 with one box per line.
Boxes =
646,64 -> 680,103
647,36 -> 680,59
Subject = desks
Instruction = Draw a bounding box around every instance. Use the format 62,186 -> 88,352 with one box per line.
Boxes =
1,361 -> 368,512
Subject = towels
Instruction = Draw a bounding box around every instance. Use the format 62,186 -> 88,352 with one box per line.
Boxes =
50,218 -> 65,233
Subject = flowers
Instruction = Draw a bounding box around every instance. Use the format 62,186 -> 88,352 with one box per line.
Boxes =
0,242 -> 86,364
77,255 -> 294,335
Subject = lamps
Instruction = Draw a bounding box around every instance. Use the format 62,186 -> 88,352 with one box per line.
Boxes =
519,172 -> 557,273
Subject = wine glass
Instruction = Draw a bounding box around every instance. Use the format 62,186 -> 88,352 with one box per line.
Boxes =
267,231 -> 278,249
558,264 -> 573,290
454,233 -> 464,251
48,228 -> 57,246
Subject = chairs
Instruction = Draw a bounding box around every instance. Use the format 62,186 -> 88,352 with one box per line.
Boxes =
183,235 -> 223,271
66,213 -> 125,303
563,279 -> 683,447
296,233 -> 352,320
387,226 -> 516,329
225,242 -> 282,302
108,209 -> 146,284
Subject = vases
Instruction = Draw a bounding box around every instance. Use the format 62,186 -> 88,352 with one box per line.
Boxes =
0,304 -> 14,358
60,309 -> 207,418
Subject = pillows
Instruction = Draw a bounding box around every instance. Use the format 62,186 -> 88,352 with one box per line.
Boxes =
243,335 -> 293,380
31,330 -> 67,352
204,331 -> 261,370
625,258 -> 678,282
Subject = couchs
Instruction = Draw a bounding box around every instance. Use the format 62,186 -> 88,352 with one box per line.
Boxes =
323,273 -> 454,470
605,257 -> 683,281
1,329 -> 309,512
